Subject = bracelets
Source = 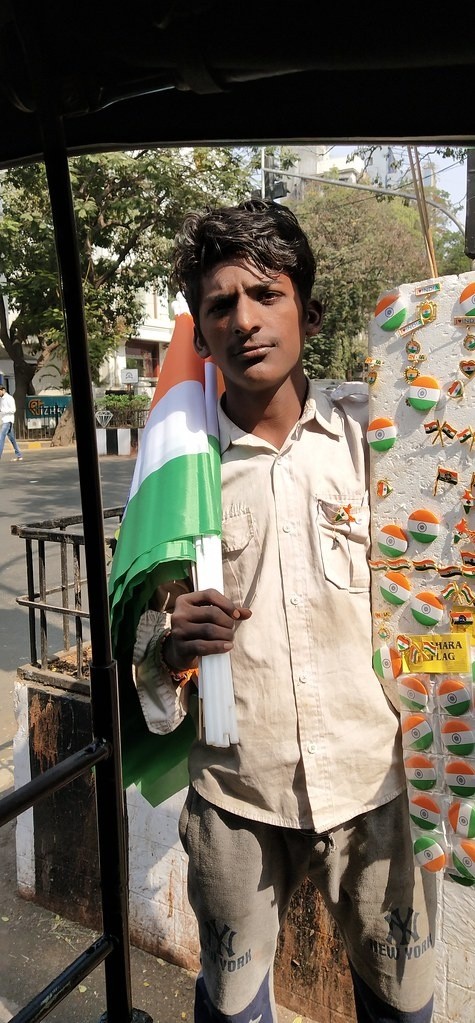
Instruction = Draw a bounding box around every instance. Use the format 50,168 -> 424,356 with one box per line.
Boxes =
157,628 -> 195,680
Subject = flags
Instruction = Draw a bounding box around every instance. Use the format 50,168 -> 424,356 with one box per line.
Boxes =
109,312 -> 226,807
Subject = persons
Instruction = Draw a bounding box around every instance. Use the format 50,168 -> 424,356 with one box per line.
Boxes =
0,384 -> 24,462
122,200 -> 436,1022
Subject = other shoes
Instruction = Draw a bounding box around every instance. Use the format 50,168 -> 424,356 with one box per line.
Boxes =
14,457 -> 23,461
